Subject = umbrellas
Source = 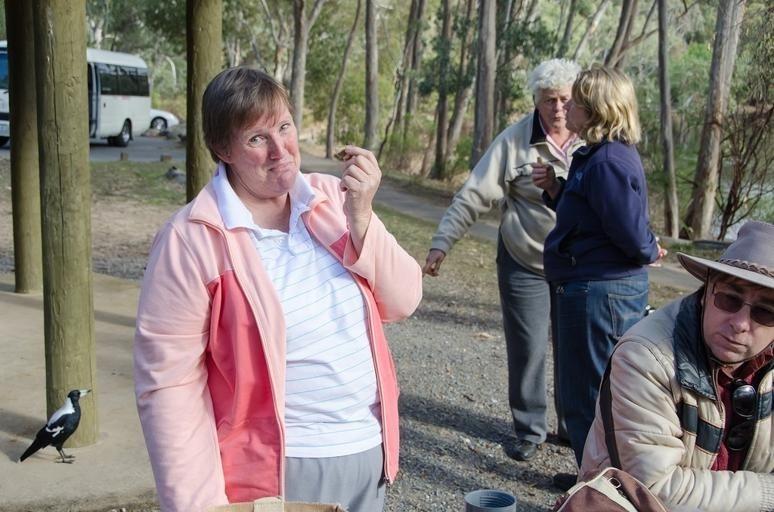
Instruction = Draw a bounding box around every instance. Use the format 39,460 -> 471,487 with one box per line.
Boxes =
513,438 -> 539,461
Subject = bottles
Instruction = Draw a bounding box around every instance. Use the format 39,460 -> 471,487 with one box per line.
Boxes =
672,217 -> 774,292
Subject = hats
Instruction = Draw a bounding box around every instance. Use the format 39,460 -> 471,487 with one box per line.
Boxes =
147,107 -> 180,137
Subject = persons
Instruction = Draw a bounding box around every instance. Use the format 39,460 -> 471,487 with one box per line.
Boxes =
576,220 -> 774,512
132,69 -> 423,512
531,65 -> 668,491
422,57 -> 588,461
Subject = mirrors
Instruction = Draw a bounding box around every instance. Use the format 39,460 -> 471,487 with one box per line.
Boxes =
19,388 -> 93,465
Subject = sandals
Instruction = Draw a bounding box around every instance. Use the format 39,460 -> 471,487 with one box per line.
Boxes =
0,40 -> 152,150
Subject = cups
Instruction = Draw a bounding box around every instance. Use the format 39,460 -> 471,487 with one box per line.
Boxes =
724,376 -> 758,454
705,286 -> 774,328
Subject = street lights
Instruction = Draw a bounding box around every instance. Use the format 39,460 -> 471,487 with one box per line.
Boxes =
548,466 -> 669,512
